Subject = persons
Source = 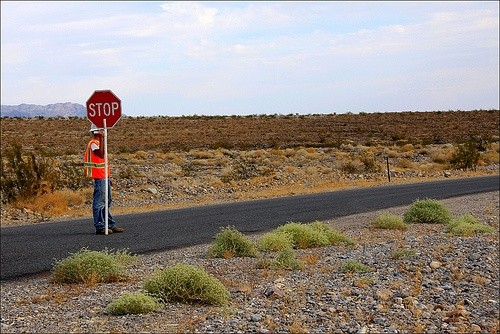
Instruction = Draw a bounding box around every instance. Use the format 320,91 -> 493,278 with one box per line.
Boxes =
83,122 -> 124,234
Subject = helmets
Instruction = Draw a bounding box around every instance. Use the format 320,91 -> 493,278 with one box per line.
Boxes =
89,123 -> 104,132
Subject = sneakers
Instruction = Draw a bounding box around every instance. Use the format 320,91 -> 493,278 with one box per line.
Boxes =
111,227 -> 124,232
95,228 -> 113,234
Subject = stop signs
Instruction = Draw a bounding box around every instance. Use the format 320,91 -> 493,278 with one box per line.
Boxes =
85,89 -> 122,129
